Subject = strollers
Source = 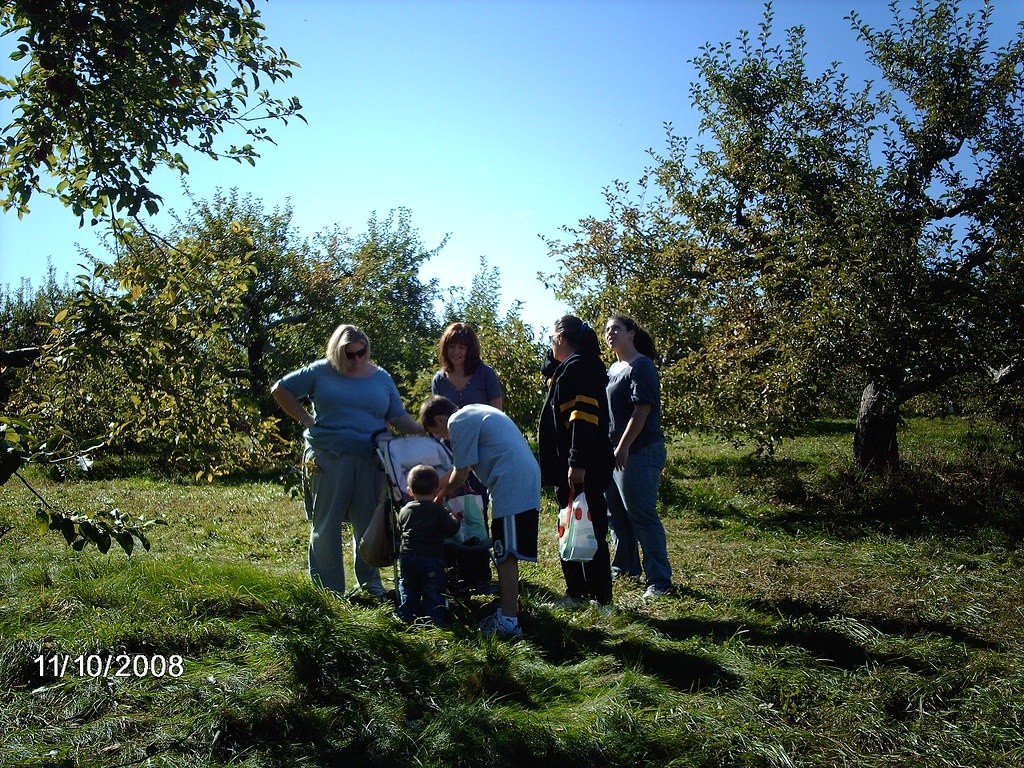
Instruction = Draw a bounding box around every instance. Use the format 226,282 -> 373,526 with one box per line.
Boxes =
370,426 -> 521,628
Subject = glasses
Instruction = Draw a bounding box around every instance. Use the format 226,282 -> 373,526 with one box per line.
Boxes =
345,345 -> 366,360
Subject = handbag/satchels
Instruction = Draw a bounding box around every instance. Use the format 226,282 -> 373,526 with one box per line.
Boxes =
557,480 -> 598,561
359,501 -> 401,567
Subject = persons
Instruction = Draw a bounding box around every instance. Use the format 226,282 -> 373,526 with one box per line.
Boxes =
394,464 -> 463,622
533,315 -> 672,622
419,394 -> 542,638
431,322 -> 502,538
269,323 -> 427,592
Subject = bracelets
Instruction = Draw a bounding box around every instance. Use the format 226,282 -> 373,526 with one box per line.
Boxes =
300,413 -> 310,426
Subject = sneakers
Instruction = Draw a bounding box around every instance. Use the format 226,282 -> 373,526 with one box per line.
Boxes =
475,608 -> 523,639
643,584 -> 673,599
611,572 -> 640,581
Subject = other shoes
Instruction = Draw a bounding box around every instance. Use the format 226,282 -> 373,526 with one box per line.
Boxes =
583,601 -> 613,617
541,595 -> 579,607
